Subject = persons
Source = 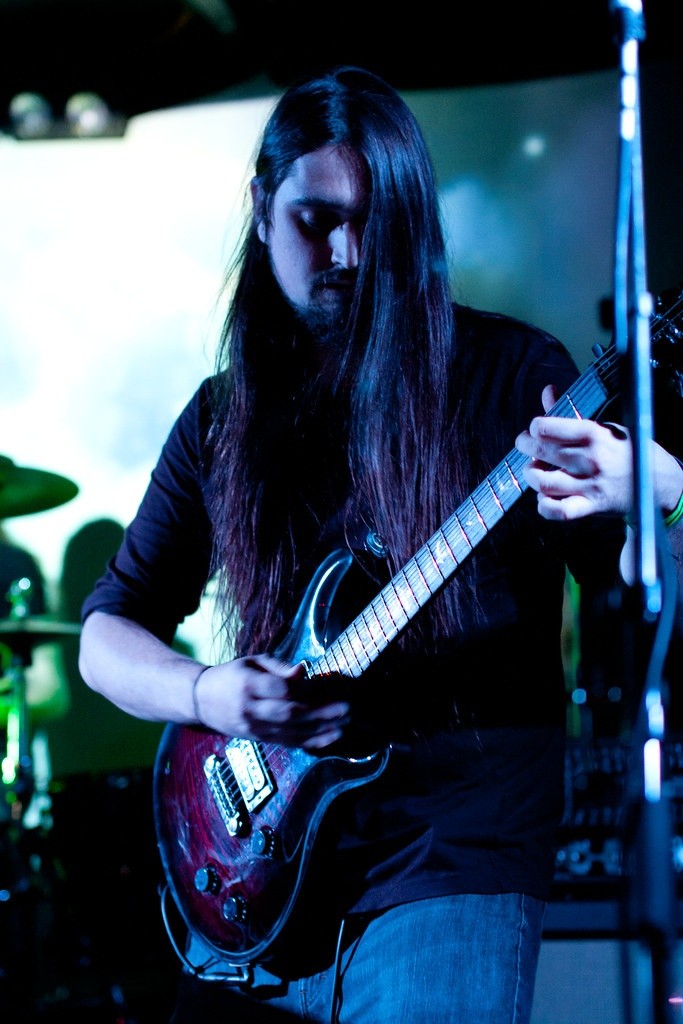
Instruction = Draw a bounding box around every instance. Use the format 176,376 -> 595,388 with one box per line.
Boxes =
79,66 -> 683,1024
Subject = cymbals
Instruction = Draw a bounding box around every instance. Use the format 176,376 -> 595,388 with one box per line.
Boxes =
0,454 -> 77,521
0,613 -> 82,643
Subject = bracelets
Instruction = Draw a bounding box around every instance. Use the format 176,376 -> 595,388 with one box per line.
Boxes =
192,665 -> 212,728
624,455 -> 683,531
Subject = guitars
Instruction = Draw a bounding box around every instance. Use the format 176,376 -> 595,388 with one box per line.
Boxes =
151,292 -> 683,966
